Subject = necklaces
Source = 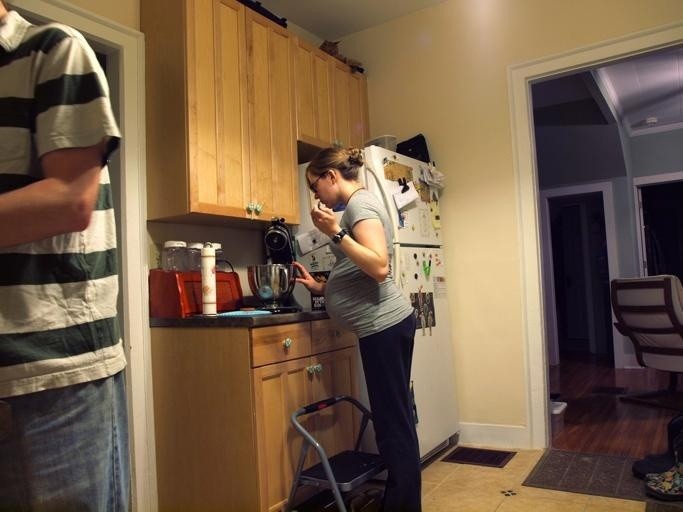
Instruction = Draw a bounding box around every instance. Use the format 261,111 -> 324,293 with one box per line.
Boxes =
344,187 -> 365,207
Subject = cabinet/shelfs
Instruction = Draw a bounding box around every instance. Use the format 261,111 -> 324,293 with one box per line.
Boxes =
151,317 -> 369,512
139,0 -> 300,227
291,34 -> 370,155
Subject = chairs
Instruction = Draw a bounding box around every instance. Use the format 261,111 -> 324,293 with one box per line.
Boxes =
291,392 -> 386,510
611,272 -> 682,408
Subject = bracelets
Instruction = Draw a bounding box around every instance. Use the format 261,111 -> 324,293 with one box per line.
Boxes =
332,230 -> 347,244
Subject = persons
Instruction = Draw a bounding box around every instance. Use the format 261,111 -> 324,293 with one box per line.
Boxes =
1,4 -> 137,510
287,144 -> 422,511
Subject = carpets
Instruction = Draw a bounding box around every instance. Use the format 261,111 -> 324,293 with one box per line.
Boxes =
521,448 -> 682,512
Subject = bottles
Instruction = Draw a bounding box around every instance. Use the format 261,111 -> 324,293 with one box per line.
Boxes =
162,240 -> 187,272
212,242 -> 225,271
200,247 -> 217,315
187,242 -> 204,272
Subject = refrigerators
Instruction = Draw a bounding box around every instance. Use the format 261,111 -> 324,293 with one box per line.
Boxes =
292,144 -> 461,467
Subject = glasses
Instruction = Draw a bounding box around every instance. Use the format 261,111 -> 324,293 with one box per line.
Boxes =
309,169 -> 331,193
